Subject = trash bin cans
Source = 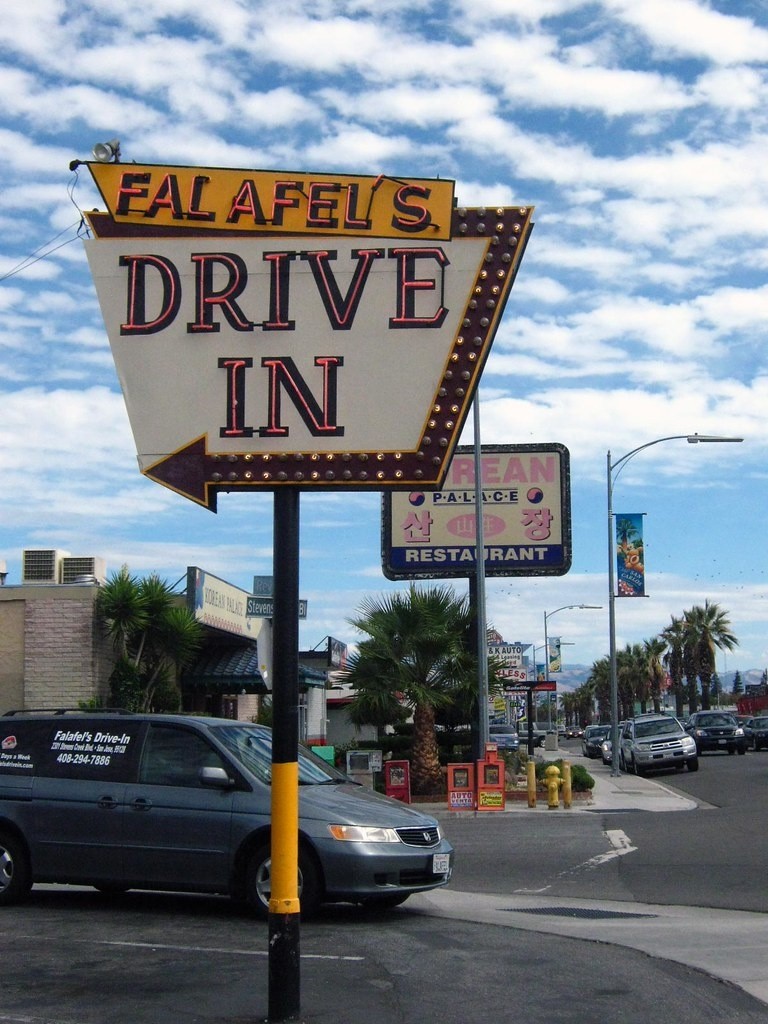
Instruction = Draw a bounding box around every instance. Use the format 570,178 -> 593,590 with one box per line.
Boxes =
545,730 -> 558,750
311,745 -> 336,767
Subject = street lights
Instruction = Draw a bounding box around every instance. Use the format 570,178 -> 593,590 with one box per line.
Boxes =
531,642 -> 577,722
606,434 -> 745,777
543,603 -> 604,729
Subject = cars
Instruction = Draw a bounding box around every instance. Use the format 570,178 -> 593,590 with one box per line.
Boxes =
742,716 -> 768,751
734,714 -> 754,729
558,720 -> 624,764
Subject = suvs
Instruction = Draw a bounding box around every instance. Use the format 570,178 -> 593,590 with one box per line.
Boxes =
0,704 -> 456,924
518,720 -> 561,747
617,711 -> 699,777
685,710 -> 746,756
490,722 -> 520,752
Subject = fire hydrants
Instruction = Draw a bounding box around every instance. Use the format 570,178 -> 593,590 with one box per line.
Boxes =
538,764 -> 566,811
